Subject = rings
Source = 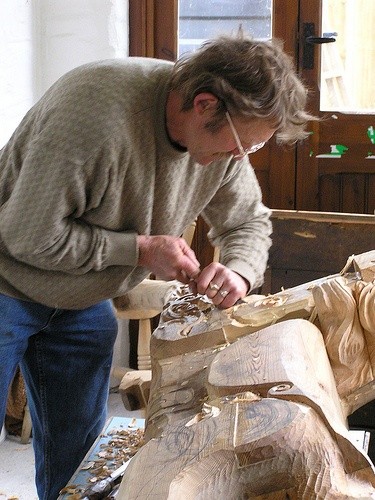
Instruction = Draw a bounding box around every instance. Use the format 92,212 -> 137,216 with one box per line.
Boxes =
219,291 -> 229,297
210,282 -> 220,290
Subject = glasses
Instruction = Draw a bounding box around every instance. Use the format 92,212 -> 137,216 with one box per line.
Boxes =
211,90 -> 265,159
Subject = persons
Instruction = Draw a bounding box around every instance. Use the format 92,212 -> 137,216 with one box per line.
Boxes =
0,23 -> 331,500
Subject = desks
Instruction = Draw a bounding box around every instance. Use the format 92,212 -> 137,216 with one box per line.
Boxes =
57,417 -> 370,499
